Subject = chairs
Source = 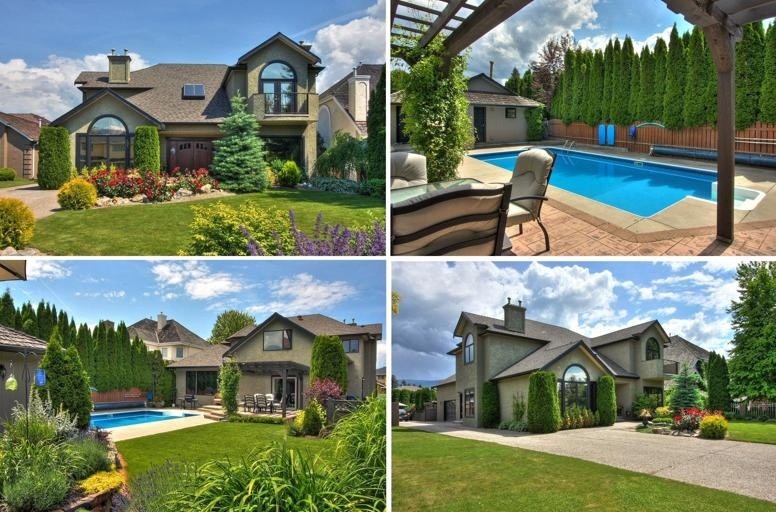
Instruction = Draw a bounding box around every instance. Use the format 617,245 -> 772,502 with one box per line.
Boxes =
389,151 -> 429,188
391,184 -> 511,255
504,146 -> 557,252
244,392 -> 291,413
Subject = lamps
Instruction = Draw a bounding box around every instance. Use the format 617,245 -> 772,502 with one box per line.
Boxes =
4,344 -> 46,453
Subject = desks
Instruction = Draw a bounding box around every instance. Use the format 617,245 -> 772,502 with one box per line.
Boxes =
391,176 -> 484,207
